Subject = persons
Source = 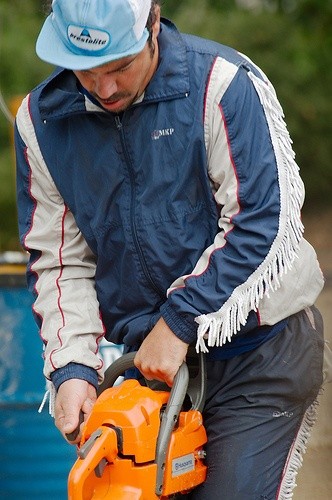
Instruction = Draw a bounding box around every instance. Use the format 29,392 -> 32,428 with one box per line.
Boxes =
12,0 -> 329,500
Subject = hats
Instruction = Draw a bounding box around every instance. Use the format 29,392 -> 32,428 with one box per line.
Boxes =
35,0 -> 152,71
